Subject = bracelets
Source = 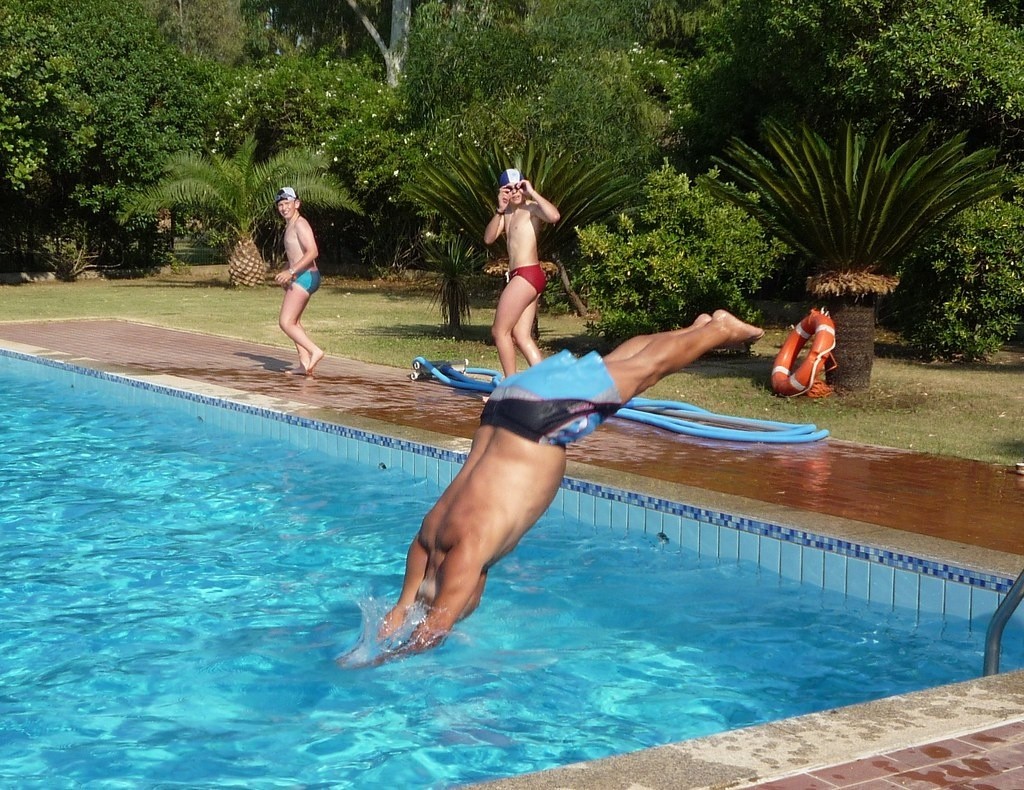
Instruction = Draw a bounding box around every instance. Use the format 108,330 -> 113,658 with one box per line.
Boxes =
288,268 -> 294,275
495,206 -> 506,215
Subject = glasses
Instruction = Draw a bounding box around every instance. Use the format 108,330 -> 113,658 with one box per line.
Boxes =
505,183 -> 522,194
275,193 -> 297,201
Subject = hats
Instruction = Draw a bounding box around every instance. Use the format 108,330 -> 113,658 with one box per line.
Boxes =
499,168 -> 525,186
275,187 -> 297,203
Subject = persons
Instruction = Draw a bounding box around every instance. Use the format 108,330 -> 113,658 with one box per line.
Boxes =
334,309 -> 766,671
274,186 -> 325,375
483,168 -> 560,379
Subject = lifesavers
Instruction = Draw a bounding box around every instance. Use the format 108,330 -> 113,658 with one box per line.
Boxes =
770,313 -> 836,397
